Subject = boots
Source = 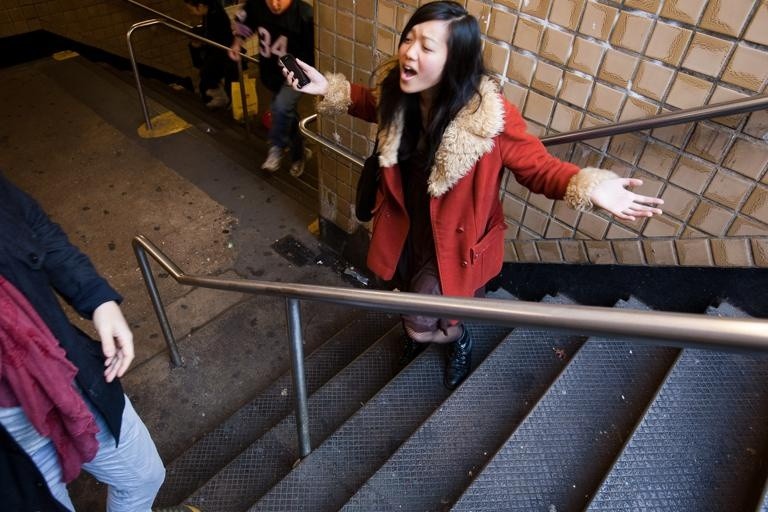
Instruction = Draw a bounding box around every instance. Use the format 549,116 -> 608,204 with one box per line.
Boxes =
206,84 -> 230,107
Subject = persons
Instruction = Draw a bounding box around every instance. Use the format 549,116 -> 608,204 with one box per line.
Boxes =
277,1 -> 667,391
185,0 -> 241,109
0,172 -> 170,511
227,0 -> 315,178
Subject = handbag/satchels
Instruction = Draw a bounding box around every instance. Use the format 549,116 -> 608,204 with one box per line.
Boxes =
354,127 -> 383,223
231,72 -> 258,122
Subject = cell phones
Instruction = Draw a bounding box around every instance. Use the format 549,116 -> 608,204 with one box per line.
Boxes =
279,53 -> 311,89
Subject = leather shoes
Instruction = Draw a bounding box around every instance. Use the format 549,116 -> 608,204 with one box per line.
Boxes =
445,322 -> 474,391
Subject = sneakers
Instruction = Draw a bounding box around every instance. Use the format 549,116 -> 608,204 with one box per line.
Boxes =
289,147 -> 312,177
261,145 -> 290,172
